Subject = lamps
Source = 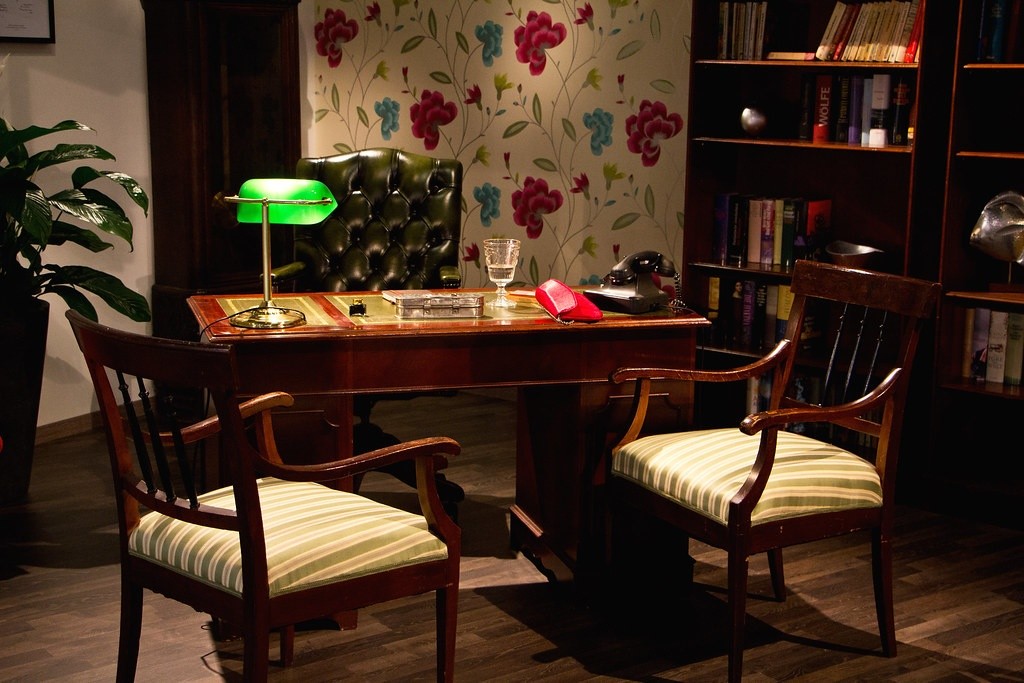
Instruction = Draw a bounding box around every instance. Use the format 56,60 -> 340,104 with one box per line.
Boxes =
222,179 -> 338,328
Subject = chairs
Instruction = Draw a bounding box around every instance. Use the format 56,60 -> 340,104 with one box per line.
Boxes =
604,259 -> 942,683
65,308 -> 461,683
260,147 -> 465,522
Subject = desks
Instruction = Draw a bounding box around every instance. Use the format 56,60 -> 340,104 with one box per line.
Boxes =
185,285 -> 712,642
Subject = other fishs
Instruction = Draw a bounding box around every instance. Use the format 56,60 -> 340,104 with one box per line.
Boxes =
969,191 -> 1024,266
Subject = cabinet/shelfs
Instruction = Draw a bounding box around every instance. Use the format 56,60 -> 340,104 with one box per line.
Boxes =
681,0 -> 1024,532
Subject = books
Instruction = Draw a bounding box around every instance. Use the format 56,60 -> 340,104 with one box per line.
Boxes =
708,0 -> 1024,448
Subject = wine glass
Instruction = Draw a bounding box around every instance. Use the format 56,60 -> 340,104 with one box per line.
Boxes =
484,238 -> 520,306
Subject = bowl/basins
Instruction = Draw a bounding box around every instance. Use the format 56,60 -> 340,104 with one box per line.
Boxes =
827,241 -> 884,271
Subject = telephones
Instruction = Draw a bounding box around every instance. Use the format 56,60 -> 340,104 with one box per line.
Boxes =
583,251 -> 676,314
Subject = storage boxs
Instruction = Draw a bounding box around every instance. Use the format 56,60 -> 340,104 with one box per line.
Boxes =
396,293 -> 484,318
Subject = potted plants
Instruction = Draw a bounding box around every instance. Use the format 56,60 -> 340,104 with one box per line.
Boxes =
0,119 -> 152,504
